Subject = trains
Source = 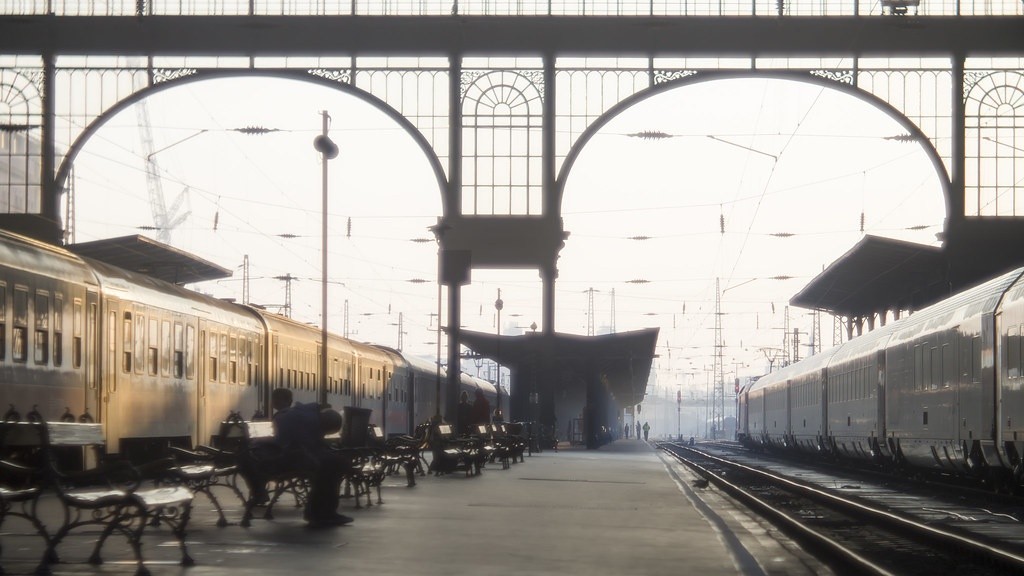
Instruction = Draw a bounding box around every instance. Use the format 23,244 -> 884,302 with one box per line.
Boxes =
706,416 -> 736,438
1,228 -> 510,482
732,261 -> 1024,497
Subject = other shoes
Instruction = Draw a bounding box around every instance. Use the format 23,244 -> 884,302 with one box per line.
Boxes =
313,514 -> 354,528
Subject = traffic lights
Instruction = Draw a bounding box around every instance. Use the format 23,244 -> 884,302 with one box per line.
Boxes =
734,377 -> 740,392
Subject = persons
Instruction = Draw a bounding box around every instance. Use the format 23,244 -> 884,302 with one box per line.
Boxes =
643,422 -> 650,441
625,423 -> 630,439
241,388 -> 353,526
470,387 -> 490,424
458,390 -> 473,429
636,421 -> 641,439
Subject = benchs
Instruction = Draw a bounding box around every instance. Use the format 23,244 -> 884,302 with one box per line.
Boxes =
0,402 -> 560,576
